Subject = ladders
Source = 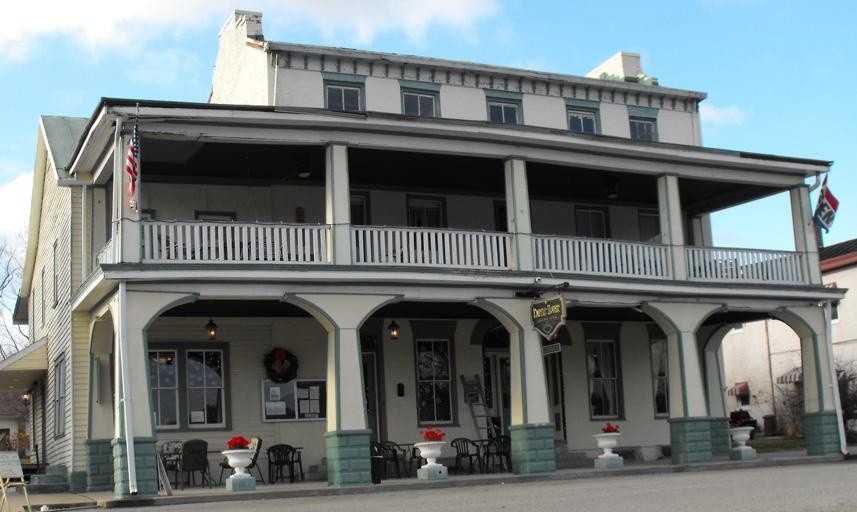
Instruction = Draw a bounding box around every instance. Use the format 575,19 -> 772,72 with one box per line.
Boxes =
461,375 -> 508,474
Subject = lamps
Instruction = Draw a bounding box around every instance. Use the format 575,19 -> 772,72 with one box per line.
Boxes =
387,305 -> 399,340
21,393 -> 31,405
204,301 -> 218,340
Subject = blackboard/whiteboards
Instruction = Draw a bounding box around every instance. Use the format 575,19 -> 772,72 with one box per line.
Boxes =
0,451 -> 24,478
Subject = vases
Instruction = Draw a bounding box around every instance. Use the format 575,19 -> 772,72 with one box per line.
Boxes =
414,441 -> 446,468
729,426 -> 755,450
592,433 -> 621,459
222,449 -> 255,477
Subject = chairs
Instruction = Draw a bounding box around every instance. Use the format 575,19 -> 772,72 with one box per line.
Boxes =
267,444 -> 296,483
450,435 -> 511,473
160,438 -> 217,491
218,436 -> 266,487
370,439 -> 409,481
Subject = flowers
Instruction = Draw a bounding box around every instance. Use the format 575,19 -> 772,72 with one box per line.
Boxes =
728,408 -> 753,427
227,436 -> 248,449
420,424 -> 446,441
601,423 -> 619,432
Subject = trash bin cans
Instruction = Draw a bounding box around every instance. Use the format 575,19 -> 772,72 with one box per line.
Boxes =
371,455 -> 383,484
762,415 -> 775,436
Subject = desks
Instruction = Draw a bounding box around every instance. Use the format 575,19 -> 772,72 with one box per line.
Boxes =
266,446 -> 303,482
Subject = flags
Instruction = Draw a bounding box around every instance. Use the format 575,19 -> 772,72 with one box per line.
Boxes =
811,164 -> 839,237
123,99 -> 144,214
